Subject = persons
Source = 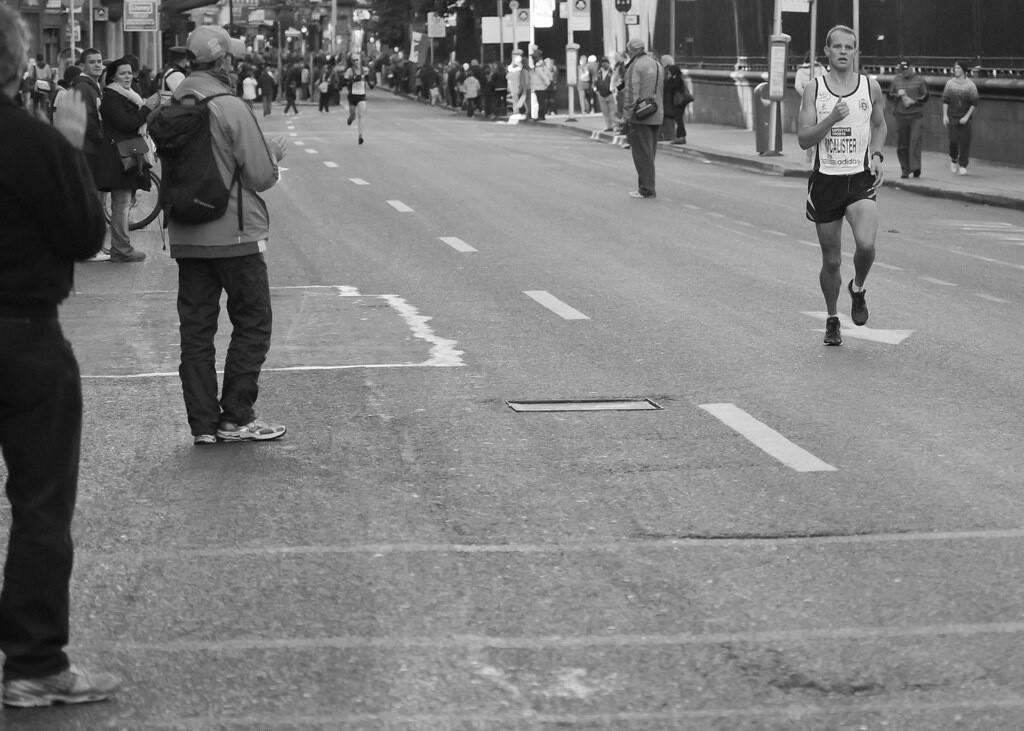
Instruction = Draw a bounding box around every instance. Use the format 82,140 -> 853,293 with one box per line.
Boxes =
232,46 -> 559,125
21,46 -> 191,263
794,50 -> 828,158
577,53 -> 693,146
889,61 -> 928,178
0,3 -> 121,708
341,53 -> 371,144
941,61 -> 979,175
619,39 -> 664,200
797,25 -> 888,346
162,24 -> 288,443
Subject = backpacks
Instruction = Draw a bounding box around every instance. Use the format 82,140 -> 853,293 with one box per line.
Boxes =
146,92 -> 243,230
158,69 -> 175,106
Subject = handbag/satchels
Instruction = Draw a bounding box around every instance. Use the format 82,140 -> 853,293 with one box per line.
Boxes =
673,85 -> 694,108
115,135 -> 153,181
634,97 -> 658,121
319,82 -> 328,93
34,80 -> 51,95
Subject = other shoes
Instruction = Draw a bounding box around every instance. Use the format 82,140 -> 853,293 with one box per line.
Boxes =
604,128 -> 612,131
111,251 -> 147,262
358,136 -> 363,144
629,191 -> 644,198
823,317 -> 842,346
847,279 -> 868,326
900,168 -> 920,178
951,161 -> 968,175
671,136 -> 686,144
86,251 -> 110,261
347,119 -> 352,125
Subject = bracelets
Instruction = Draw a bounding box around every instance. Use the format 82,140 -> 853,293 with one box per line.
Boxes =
872,151 -> 884,162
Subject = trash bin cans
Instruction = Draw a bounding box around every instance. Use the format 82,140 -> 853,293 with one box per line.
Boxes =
754,83 -> 786,156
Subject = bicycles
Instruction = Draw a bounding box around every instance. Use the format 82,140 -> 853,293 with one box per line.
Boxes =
102,167 -> 163,233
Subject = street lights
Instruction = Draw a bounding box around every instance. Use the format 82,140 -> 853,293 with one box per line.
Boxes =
509,0 -> 520,51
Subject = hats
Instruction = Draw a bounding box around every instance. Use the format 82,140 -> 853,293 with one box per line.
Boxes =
900,59 -> 911,69
600,56 -> 609,61
186,25 -> 246,64
168,46 -> 188,63
955,60 -> 966,71
121,53 -> 140,73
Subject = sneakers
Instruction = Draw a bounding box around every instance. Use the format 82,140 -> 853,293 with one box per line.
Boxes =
216,419 -> 285,443
1,665 -> 121,707
194,431 -> 218,444
